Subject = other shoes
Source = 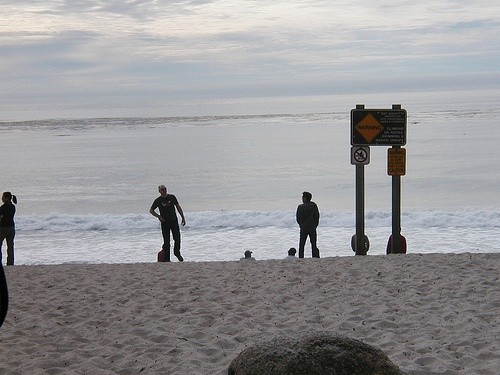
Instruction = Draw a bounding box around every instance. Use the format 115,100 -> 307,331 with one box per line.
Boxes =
174,253 -> 183,261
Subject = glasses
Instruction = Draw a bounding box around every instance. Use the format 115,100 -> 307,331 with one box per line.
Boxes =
161,188 -> 166,191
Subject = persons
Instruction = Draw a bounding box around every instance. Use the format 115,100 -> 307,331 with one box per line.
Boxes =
0,192 -> 18,266
285,248 -> 297,259
296,191 -> 320,258
0,261 -> 9,328
149,185 -> 185,262
244,250 -> 252,259
